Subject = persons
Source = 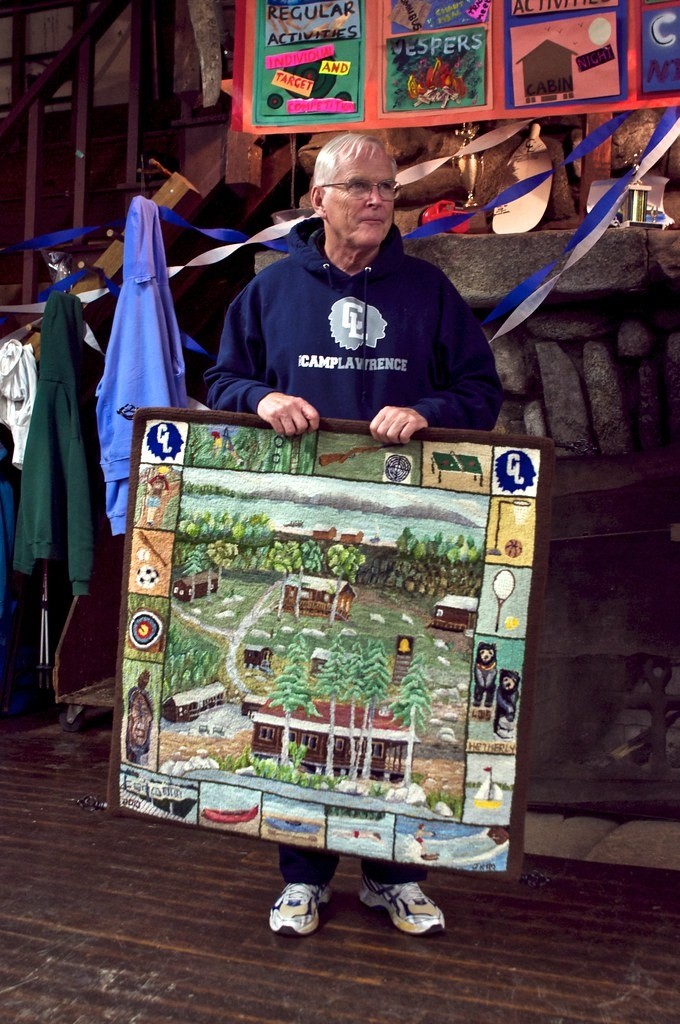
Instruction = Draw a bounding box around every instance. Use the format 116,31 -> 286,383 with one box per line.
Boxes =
211,133 -> 503,934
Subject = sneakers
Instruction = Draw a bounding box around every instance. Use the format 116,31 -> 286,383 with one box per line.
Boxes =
270,882 -> 329,935
358,875 -> 445,936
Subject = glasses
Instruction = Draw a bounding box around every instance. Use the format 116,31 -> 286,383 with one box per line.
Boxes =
321,179 -> 401,201
